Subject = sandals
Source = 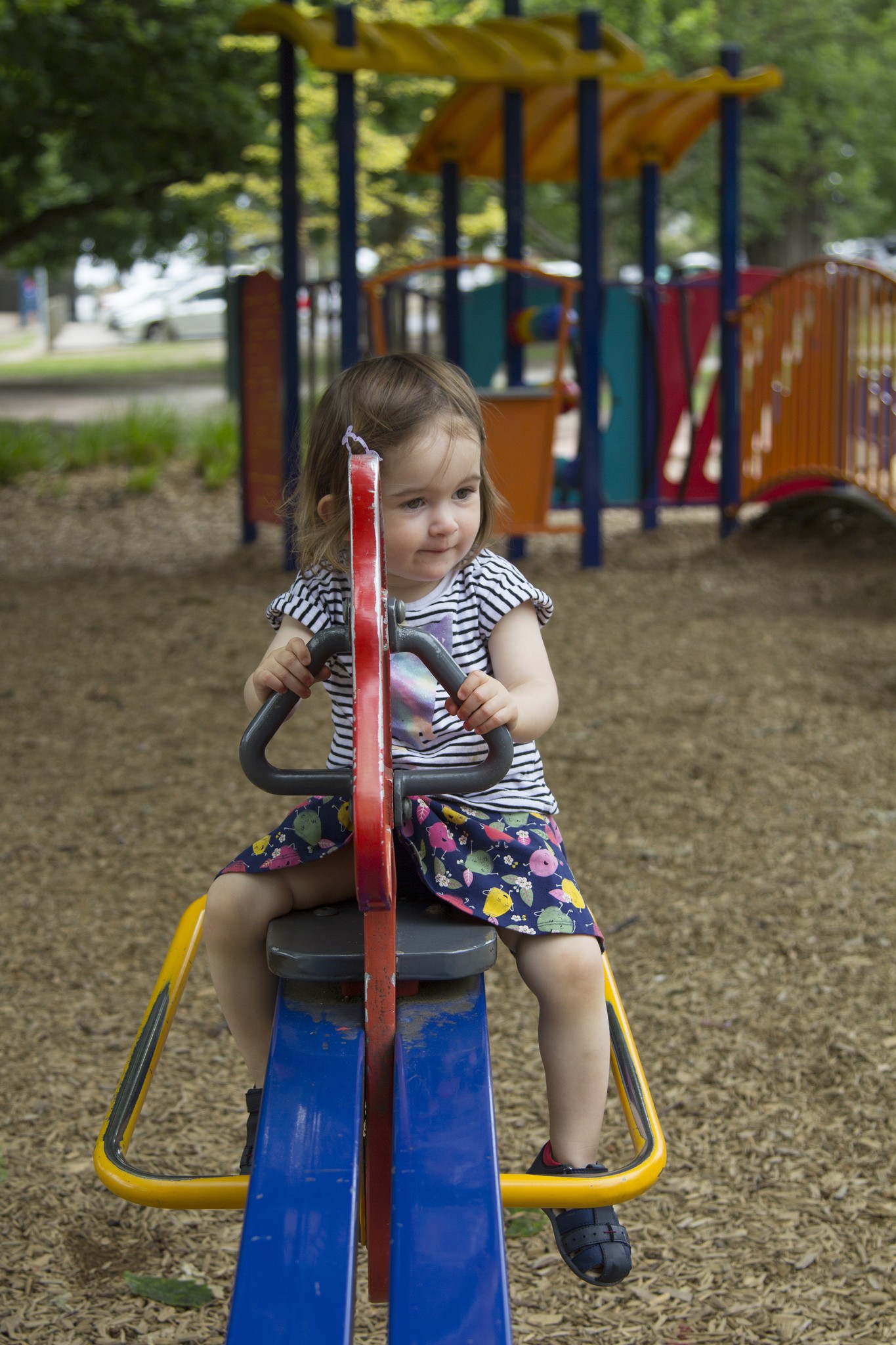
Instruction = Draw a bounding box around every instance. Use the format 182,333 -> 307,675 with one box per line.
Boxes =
525,1139 -> 633,1287
239,1084 -> 263,1175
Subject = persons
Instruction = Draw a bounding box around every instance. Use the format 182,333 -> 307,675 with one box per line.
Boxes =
202,351 -> 633,1285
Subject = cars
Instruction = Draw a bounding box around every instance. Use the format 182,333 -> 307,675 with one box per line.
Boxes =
104,265 -> 327,342
